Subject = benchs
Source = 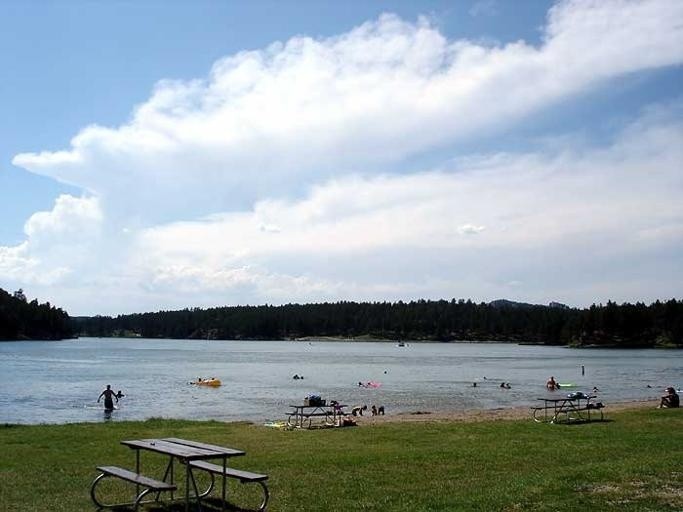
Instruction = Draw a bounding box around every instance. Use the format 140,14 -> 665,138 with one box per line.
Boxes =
284,411 -> 351,429
530,403 -> 605,425
88,459 -> 269,512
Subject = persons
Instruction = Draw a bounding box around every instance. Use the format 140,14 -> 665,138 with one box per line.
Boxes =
115,390 -> 126,399
546,377 -> 559,391
351,404 -> 368,417
372,405 -> 378,417
655,387 -> 680,410
98,384 -> 120,410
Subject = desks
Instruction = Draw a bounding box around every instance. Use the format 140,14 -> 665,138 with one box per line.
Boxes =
289,404 -> 347,428
536,395 -> 597,424
118,436 -> 245,512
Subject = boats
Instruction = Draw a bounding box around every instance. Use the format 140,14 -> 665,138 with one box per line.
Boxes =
196,380 -> 221,386
